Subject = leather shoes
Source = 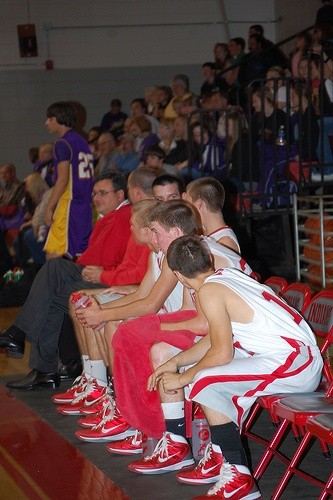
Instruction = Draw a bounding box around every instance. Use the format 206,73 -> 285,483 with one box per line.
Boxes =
7,369 -> 60,390
58,362 -> 83,379
0,329 -> 25,359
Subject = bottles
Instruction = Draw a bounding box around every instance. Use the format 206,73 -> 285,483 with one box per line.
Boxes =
278,125 -> 285,143
6,269 -> 24,281
71,292 -> 108,331
191,403 -> 212,461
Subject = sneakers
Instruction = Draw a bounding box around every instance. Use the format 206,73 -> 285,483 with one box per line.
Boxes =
51,370 -> 138,442
128,431 -> 195,474
175,441 -> 227,485
191,462 -> 262,500
107,429 -> 148,455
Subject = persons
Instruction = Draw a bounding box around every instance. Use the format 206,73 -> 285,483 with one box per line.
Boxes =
88,73 -> 247,191
251,0 -> 333,184
200,25 -> 290,111
0,144 -> 52,265
0,170 -> 323,500
44,101 -> 91,261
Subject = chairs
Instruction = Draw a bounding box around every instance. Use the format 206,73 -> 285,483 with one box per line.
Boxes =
238,271 -> 333,500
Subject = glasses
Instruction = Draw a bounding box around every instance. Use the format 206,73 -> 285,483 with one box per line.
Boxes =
91,188 -> 124,199
